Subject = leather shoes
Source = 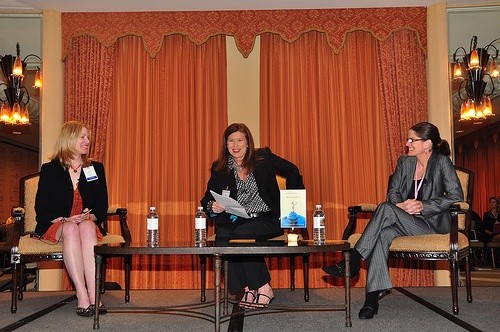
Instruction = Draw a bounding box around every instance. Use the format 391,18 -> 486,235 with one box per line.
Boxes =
322,261 -> 355,278
359,300 -> 379,319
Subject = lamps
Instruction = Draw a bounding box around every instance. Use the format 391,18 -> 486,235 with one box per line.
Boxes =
452,36 -> 500,121
0,42 -> 41,126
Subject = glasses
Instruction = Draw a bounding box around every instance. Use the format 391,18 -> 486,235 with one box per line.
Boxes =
406,137 -> 431,143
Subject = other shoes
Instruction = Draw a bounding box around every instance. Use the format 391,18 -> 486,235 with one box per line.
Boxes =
76,304 -> 93,316
91,302 -> 107,314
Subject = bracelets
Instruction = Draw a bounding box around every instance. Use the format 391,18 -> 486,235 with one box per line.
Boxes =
207,200 -> 212,213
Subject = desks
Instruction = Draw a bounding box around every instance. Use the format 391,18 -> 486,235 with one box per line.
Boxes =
93,240 -> 351,332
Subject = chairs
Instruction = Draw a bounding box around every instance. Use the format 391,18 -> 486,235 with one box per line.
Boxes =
199,219 -> 310,303
342,165 -> 500,315
0,172 -> 132,314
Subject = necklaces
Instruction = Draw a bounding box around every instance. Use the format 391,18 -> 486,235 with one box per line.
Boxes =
69,163 -> 82,173
238,166 -> 245,172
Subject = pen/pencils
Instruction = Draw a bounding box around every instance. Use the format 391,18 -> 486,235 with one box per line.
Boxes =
84,209 -> 92,215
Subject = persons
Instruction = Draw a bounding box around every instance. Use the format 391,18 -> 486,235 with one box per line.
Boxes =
33,120 -> 108,317
321,122 -> 465,319
470,196 -> 500,270
200,123 -> 307,309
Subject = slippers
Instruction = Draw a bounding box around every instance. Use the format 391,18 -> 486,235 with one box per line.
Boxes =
248,292 -> 275,310
238,291 -> 256,310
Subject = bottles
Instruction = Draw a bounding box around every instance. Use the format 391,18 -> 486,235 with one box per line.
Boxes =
146,207 -> 159,247
312,205 -> 326,245
194,206 -> 207,247
